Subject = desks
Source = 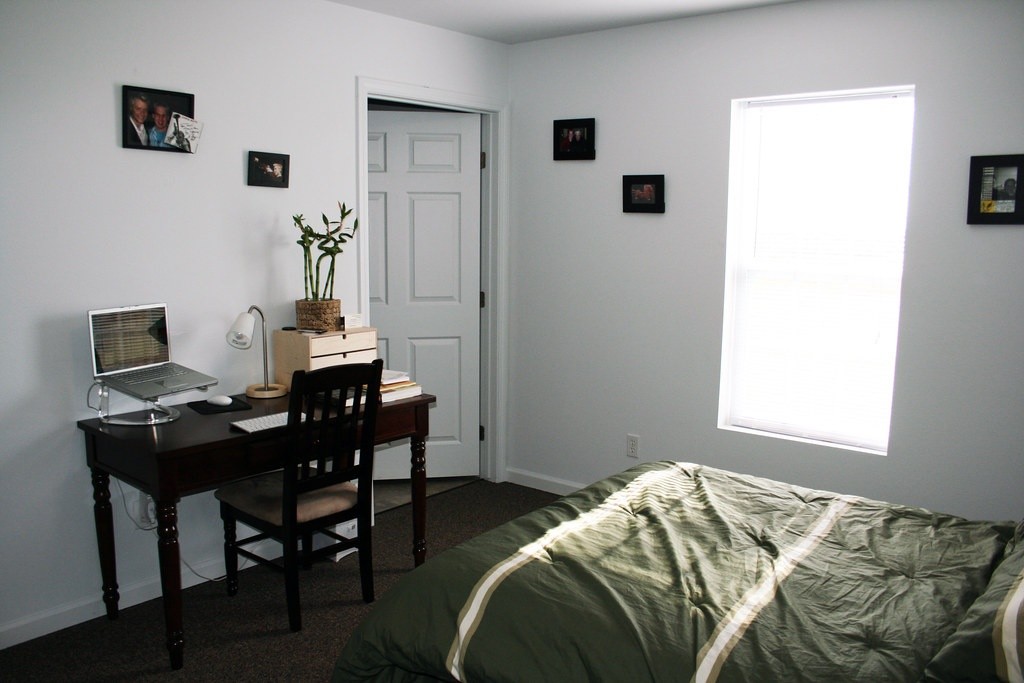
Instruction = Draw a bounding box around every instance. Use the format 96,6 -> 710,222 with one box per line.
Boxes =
75,389 -> 436,669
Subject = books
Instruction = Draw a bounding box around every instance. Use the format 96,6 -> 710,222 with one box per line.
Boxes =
317,386 -> 376,408
350,369 -> 422,404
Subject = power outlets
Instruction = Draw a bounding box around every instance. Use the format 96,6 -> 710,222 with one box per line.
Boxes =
626,433 -> 640,458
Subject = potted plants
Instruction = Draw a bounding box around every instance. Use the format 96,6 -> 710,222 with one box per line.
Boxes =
293,201 -> 359,333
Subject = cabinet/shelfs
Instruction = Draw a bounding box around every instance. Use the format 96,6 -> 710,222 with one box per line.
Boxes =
272,326 -> 378,395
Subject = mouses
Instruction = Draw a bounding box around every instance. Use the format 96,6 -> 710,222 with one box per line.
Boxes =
208,395 -> 232,405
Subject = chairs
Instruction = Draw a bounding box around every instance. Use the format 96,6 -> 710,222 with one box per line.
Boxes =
213,357 -> 378,634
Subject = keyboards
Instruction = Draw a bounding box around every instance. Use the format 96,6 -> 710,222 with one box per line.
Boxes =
229,411 -> 315,434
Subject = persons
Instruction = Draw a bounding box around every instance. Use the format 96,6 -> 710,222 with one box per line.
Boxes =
560,129 -> 575,153
147,101 -> 175,147
632,184 -> 656,203
571,131 -> 586,153
271,163 -> 283,184
124,91 -> 151,147
992,174 -> 1016,200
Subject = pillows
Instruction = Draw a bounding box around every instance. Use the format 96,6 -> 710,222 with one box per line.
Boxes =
919,548 -> 1024,682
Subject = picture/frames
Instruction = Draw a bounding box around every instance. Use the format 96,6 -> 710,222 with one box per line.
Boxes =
966,155 -> 1024,225
247,151 -> 290,190
622,174 -> 665,213
553,118 -> 595,161
120,83 -> 195,152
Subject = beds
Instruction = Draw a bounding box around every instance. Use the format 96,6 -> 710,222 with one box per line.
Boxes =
328,460 -> 1024,683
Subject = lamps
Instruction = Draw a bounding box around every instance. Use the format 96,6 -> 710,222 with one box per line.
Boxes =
224,304 -> 289,399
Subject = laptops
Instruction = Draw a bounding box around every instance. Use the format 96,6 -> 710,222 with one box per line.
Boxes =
88,302 -> 219,402
